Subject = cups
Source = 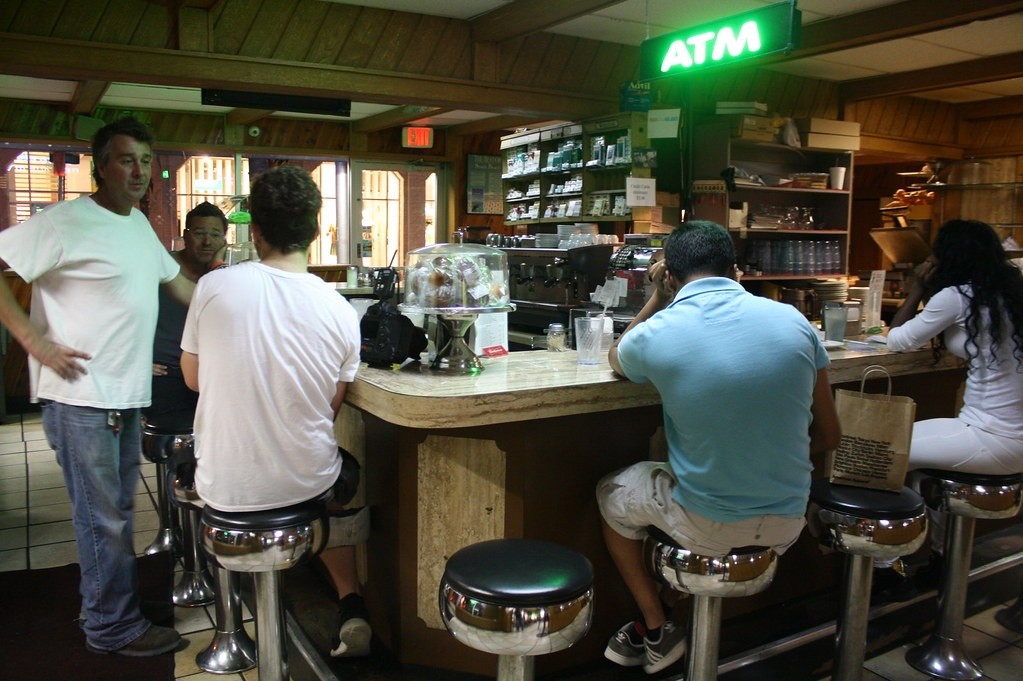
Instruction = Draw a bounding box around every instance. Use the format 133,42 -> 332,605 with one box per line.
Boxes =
486,233 -> 533,248
574,317 -> 604,366
347,266 -> 357,289
559,234 -> 619,249
828,166 -> 846,190
824,304 -> 848,343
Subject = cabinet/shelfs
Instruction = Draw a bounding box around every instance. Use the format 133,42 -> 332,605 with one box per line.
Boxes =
499,109 -> 655,239
688,114 -> 854,323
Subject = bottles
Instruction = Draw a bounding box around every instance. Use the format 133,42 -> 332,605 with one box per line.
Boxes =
744,239 -> 841,276
358,267 -> 405,289
546,322 -> 566,352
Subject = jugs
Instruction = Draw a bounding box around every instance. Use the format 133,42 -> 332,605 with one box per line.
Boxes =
781,286 -> 818,321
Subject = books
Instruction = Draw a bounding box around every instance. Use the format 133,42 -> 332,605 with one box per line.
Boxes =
716,100 -> 767,116
733,177 -> 762,186
794,173 -> 829,189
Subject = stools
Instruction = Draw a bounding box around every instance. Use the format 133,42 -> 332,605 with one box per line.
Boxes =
141,420 -> 326,681
809,468 -> 932,681
438,539 -> 595,681
642,534 -> 778,681
905,466 -> 1023,681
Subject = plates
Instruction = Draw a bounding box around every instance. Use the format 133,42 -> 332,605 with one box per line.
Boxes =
811,281 -> 849,302
535,223 -> 599,248
850,288 -> 870,323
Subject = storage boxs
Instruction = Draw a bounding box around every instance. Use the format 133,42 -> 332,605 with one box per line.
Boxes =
630,101 -> 863,232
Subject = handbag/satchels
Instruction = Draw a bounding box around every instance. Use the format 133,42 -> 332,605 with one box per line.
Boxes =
824,364 -> 917,493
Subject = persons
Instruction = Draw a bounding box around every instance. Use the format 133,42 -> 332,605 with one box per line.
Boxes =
596,220 -> 843,673
881,218 -> 1023,636
178,165 -> 374,657
139,201 -> 229,427
0,115 -> 195,657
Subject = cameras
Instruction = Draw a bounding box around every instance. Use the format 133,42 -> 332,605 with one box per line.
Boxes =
359,302 -> 429,366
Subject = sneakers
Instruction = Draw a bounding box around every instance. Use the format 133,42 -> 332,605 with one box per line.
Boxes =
330,594 -> 371,660
87,624 -> 181,657
605,621 -> 642,666
643,620 -> 687,673
